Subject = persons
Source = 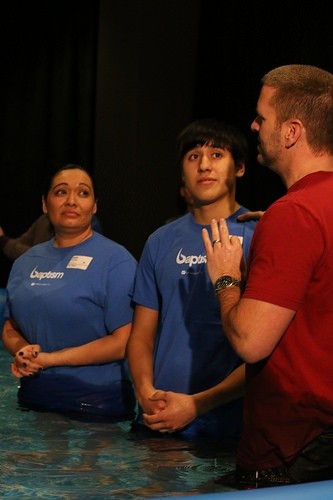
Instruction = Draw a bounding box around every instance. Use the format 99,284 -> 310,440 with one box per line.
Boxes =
0,162 -> 138,418
127,130 -> 259,438
202,65 -> 333,489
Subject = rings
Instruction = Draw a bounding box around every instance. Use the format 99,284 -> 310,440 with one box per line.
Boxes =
212,240 -> 221,246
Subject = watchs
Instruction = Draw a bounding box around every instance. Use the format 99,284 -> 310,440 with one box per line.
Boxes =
214,276 -> 241,295
